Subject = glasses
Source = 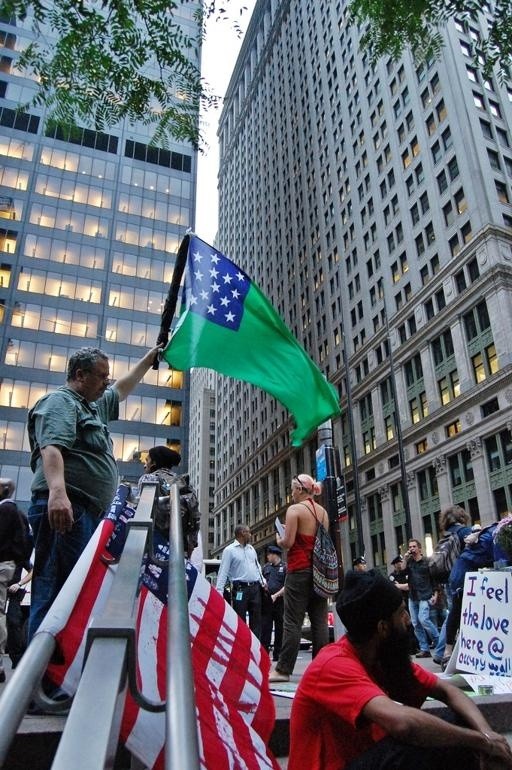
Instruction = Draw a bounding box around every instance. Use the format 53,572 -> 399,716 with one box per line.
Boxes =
292,474 -> 308,493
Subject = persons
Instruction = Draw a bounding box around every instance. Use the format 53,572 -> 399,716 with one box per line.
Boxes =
1,478 -> 34,683
261,545 -> 287,662
286,567 -> 511,769
267,475 -> 331,683
26,342 -> 167,717
215,524 -> 268,644
137,447 -> 200,559
350,505 -> 506,672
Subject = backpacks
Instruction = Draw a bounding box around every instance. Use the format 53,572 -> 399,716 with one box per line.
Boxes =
427,526 -> 466,584
298,502 -> 342,598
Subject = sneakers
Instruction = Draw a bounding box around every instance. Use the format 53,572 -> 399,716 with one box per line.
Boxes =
433,655 -> 442,665
442,656 -> 451,672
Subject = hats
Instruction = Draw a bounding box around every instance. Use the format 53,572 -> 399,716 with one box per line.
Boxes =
267,545 -> 283,554
391,555 -> 403,564
335,568 -> 402,634
149,446 -> 181,468
353,556 -> 366,564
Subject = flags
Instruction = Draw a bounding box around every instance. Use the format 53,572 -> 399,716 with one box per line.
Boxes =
32,483 -> 280,769
158,232 -> 340,450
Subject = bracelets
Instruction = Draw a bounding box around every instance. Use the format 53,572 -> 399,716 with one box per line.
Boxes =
472,732 -> 493,763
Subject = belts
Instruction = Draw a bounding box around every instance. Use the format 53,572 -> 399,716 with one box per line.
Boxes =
239,581 -> 260,587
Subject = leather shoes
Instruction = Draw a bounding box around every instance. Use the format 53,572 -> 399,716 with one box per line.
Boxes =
416,650 -> 431,658
27,687 -> 71,715
268,672 -> 290,682
